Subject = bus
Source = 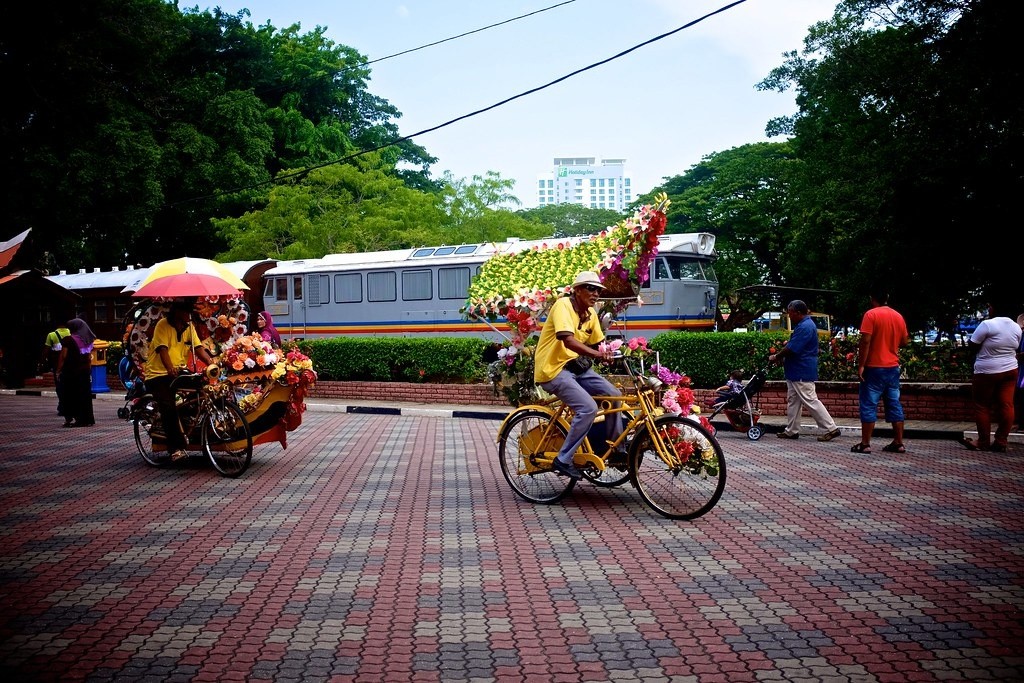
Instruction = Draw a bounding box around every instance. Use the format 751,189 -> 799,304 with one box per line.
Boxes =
262,233 -> 719,344
759,310 -> 831,341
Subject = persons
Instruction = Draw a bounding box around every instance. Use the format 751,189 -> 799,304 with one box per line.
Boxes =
957,299 -> 1023,453
1015,314 -> 1024,431
849,284 -> 910,453
37,312 -> 98,427
768,300 -> 841,442
257,311 -> 282,348
715,369 -> 744,402
143,301 -> 219,462
533,269 -> 629,481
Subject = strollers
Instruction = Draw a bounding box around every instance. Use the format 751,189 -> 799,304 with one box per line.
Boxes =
703,359 -> 780,441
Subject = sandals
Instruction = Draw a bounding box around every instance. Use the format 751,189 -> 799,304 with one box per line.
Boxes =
851,442 -> 871,454
883,441 -> 906,453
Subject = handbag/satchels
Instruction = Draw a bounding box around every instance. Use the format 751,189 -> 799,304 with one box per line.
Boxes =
563,354 -> 592,376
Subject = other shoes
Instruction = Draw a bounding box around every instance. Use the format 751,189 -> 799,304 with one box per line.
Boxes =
777,432 -> 799,439
958,437 -> 976,450
171,449 -> 186,462
817,428 -> 841,441
72,421 -> 93,427
990,442 -> 1007,453
551,457 -> 583,481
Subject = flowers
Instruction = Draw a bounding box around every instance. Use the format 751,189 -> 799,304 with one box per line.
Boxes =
122,291 -> 317,427
461,192 -> 724,477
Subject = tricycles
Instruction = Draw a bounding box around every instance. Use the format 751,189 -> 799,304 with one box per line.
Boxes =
131,365 -> 253,479
496,312 -> 727,521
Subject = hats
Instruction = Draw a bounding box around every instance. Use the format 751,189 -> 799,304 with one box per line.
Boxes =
572,271 -> 607,292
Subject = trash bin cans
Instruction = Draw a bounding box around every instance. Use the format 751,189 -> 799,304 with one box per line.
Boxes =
89,339 -> 110,392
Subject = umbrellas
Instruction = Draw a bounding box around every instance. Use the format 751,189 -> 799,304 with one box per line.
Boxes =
734,278 -> 842,294
132,271 -> 242,372
120,256 -> 251,295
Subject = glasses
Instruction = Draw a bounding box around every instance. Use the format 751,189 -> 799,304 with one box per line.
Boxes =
579,285 -> 602,293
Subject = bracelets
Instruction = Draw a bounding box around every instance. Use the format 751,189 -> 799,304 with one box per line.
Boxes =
776,354 -> 782,359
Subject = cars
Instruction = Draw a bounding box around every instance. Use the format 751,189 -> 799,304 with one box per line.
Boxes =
911,331 -> 973,348
